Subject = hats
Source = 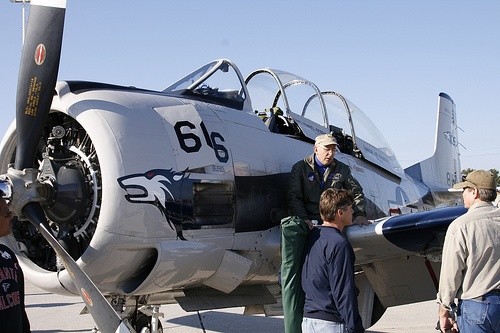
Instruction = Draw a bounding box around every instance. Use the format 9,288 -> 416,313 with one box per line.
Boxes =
453,168 -> 496,189
315,134 -> 340,146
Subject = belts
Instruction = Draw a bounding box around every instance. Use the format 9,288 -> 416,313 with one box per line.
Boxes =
489,288 -> 500,293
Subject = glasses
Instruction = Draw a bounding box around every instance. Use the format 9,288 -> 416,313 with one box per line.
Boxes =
319,145 -> 338,151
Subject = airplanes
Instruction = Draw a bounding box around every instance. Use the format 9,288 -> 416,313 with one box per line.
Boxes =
0,0 -> 469,333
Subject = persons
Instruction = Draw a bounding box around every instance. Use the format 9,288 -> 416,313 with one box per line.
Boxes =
277,134 -> 372,296
0,194 -> 32,333
300,187 -> 365,333
436,169 -> 500,333
